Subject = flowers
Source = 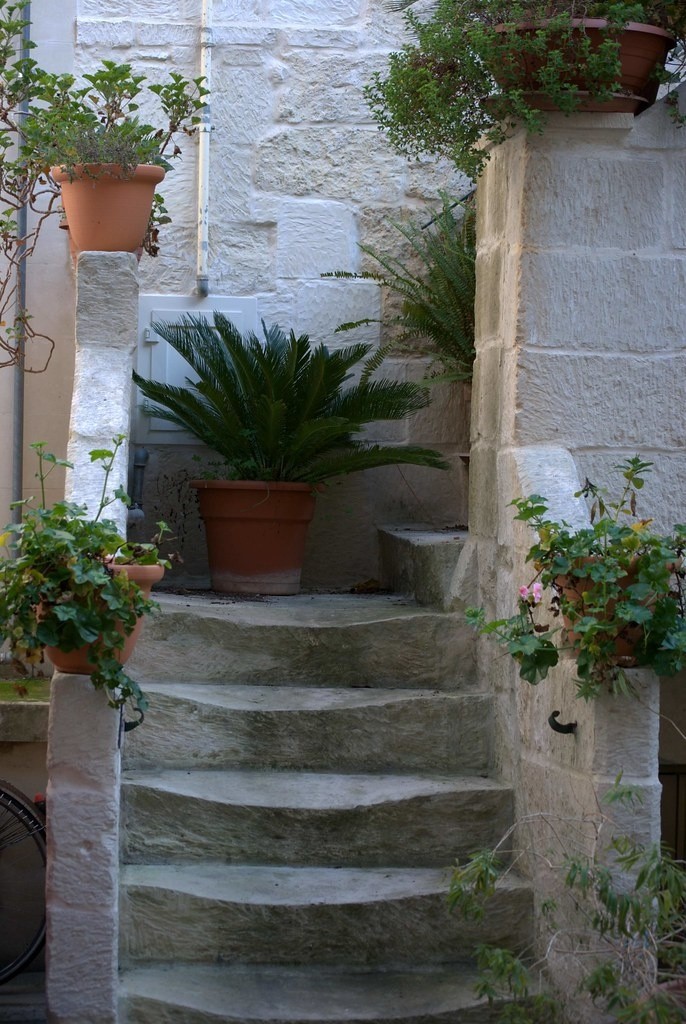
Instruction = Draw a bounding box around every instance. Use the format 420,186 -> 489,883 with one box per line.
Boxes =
462,456 -> 685,703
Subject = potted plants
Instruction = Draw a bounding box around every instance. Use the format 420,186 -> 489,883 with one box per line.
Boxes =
361,0 -> 686,183
0,57 -> 210,251
0,436 -> 184,733
131,313 -> 453,596
438,767 -> 686,1024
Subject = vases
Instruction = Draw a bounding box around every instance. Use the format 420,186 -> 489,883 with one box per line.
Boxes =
534,553 -> 683,661
